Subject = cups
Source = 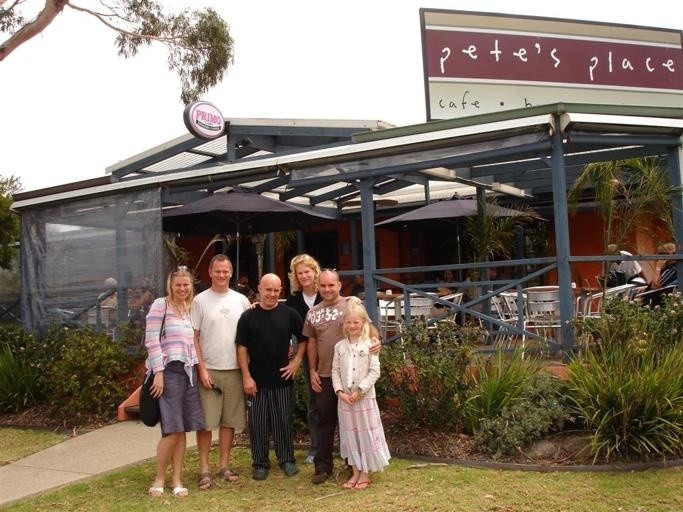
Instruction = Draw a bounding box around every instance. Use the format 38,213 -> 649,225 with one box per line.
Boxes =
571,283 -> 576,288
386,290 -> 392,295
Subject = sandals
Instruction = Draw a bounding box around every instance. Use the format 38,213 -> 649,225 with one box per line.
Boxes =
196,472 -> 213,491
216,466 -> 240,482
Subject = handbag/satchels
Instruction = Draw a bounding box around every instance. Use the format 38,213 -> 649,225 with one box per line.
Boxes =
139,369 -> 161,427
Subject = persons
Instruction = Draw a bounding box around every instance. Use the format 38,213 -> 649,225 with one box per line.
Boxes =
436,270 -> 457,295
247,254 -> 323,463
143,266 -> 206,499
190,256 -> 250,490
302,270 -> 381,482
329,301 -> 391,490
636,243 -> 678,307
237,272 -> 307,479
605,244 -> 648,284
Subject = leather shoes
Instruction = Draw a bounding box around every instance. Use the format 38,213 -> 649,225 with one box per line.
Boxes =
279,462 -> 299,477
311,471 -> 332,485
253,468 -> 269,480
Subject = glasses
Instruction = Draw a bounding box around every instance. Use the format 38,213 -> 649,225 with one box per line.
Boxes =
211,383 -> 222,396
172,265 -> 193,274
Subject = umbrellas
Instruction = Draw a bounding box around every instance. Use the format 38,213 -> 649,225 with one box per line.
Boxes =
375,191 -> 548,280
126,190 -> 336,277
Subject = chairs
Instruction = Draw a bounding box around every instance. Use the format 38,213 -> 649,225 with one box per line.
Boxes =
577,284 -> 638,358
522,285 -> 577,359
434,293 -> 463,345
634,284 -> 676,311
488,290 -> 542,356
379,299 -> 405,342
384,292 -> 442,360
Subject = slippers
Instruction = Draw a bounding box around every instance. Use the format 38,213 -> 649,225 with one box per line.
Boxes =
355,480 -> 372,490
148,487 -> 164,498
341,481 -> 354,489
172,487 -> 189,499
